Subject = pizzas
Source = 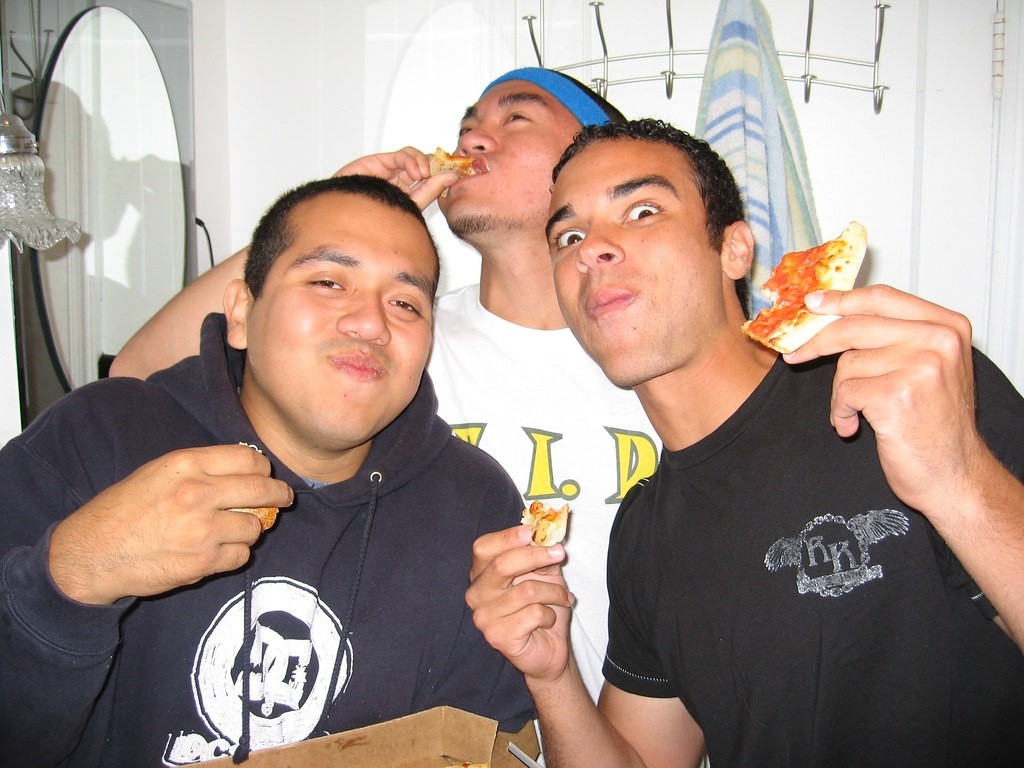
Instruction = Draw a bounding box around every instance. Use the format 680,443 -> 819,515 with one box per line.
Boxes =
429,147 -> 476,197
519,500 -> 570,548
739,221 -> 867,353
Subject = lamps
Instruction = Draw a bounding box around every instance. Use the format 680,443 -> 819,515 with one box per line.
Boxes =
0,91 -> 82,254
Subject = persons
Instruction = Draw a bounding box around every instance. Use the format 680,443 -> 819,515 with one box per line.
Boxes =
0,172 -> 545,768
462,115 -> 1024,768
100,65 -> 716,768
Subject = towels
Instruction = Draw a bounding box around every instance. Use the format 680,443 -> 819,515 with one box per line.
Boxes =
695,1 -> 824,322
